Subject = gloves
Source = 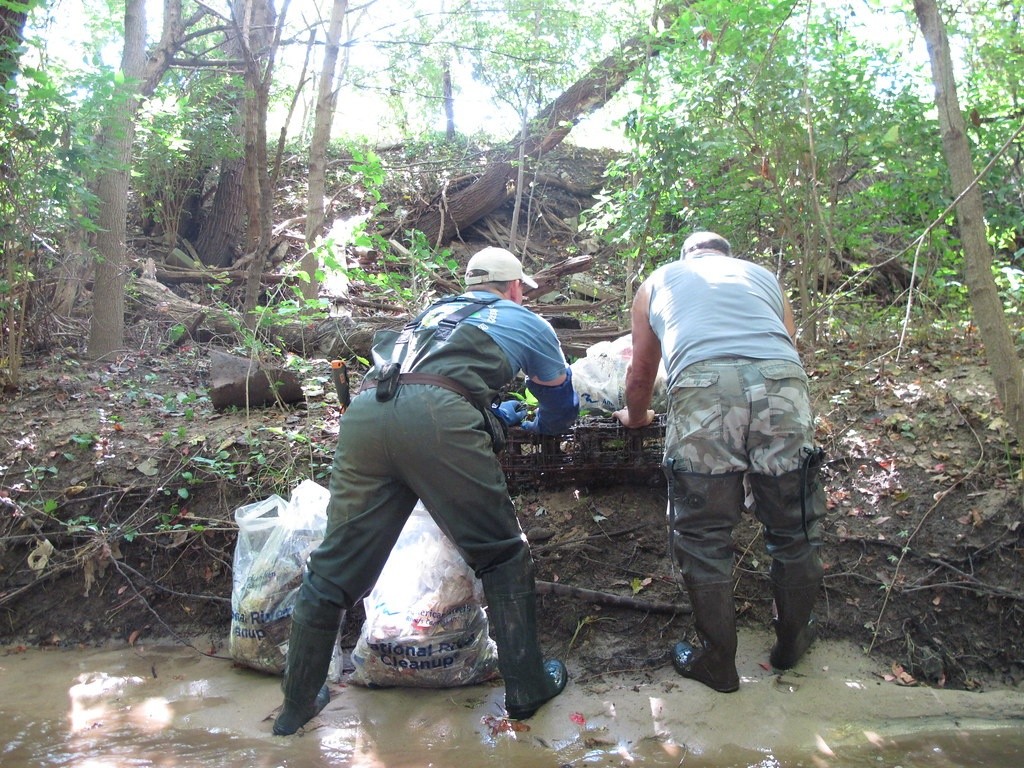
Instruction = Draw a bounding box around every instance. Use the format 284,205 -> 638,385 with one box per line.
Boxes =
491,399 -> 528,426
521,365 -> 579,436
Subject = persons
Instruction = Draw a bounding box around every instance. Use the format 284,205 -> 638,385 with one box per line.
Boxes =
613,229 -> 827,693
274,246 -> 580,738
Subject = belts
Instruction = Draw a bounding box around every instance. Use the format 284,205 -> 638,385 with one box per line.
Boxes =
356,373 -> 476,408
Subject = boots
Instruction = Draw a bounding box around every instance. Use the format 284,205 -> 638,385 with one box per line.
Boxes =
487,592 -> 567,721
671,581 -> 739,694
273,616 -> 339,736
769,578 -> 817,670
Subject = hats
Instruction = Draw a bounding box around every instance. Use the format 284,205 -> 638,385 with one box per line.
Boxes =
680,232 -> 730,258
465,247 -> 539,289
